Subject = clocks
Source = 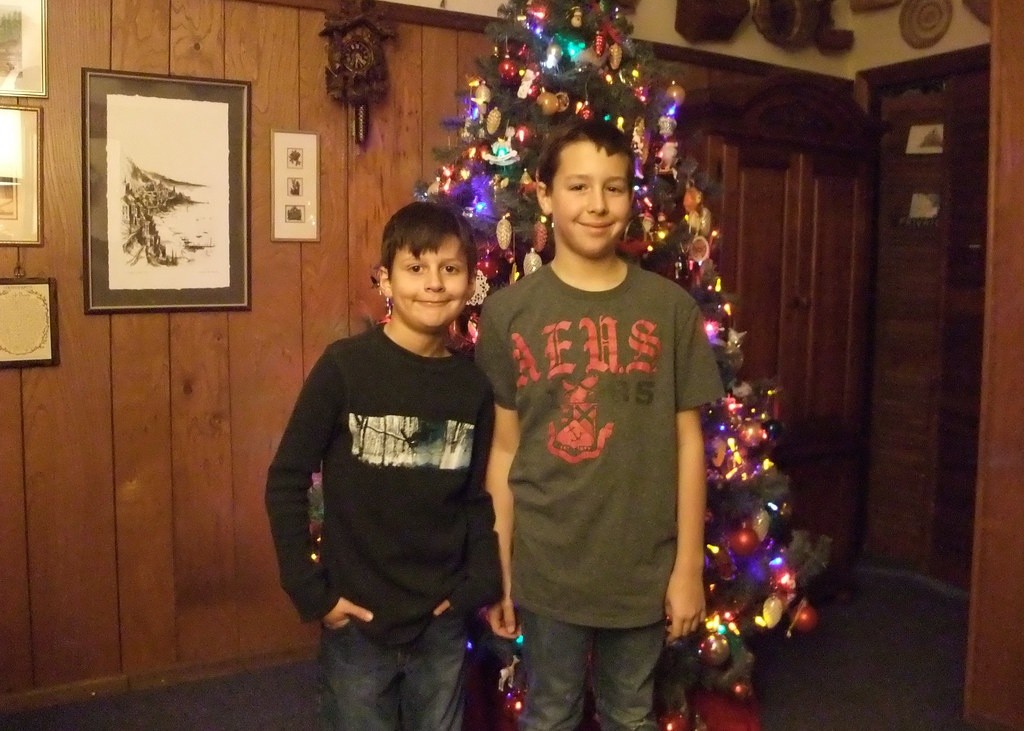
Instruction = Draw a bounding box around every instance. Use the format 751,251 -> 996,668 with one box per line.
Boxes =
317,0 -> 398,146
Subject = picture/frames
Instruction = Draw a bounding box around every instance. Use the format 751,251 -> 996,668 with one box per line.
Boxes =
0,0 -> 49,99
271,128 -> 321,242
0,276 -> 60,369
80,67 -> 252,312
0,103 -> 43,246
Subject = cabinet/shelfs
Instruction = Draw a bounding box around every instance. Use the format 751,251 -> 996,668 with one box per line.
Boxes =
672,76 -> 892,602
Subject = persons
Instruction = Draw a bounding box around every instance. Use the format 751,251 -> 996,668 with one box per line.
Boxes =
263,200 -> 505,731
474,119 -> 726,731
